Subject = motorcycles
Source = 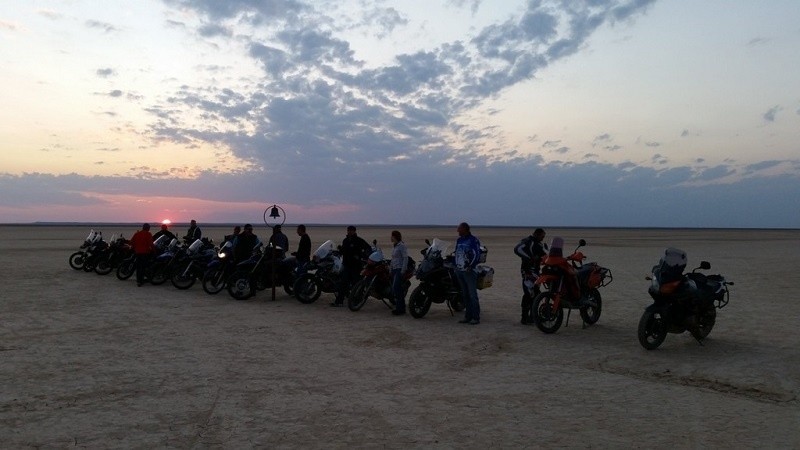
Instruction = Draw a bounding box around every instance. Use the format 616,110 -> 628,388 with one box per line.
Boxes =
408,237 -> 495,319
637,259 -> 736,350
522,236 -> 614,335
291,239 -> 365,304
67,229 -> 310,300
347,238 -> 415,312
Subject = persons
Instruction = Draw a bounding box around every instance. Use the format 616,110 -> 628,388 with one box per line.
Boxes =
329,225 -> 372,307
514,228 -> 547,326
220,224 -> 260,251
391,230 -> 409,316
152,224 -> 180,245
454,222 -> 481,326
117,223 -> 158,286
269,225 -> 288,263
187,220 -> 201,245
291,224 -> 312,298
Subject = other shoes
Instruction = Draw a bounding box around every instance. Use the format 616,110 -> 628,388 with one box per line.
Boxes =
459,319 -> 469,323
392,309 -> 406,316
330,302 -> 344,307
521,316 -> 536,326
469,319 -> 480,325
